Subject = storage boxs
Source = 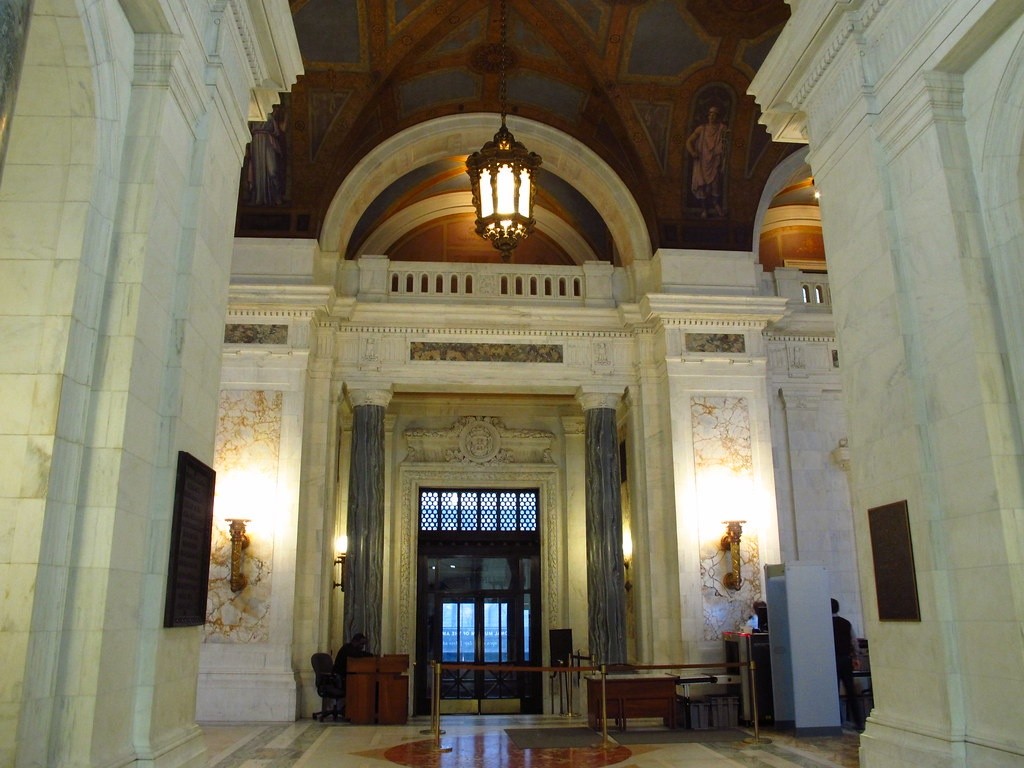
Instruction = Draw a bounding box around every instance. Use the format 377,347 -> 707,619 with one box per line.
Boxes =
686,693 -> 741,730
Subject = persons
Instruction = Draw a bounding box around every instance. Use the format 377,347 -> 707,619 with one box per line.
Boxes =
334,633 -> 373,722
830,598 -> 865,733
746,602 -> 767,632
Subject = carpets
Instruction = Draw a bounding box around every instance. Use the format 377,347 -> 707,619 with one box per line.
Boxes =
607,730 -> 755,745
505,726 -> 606,750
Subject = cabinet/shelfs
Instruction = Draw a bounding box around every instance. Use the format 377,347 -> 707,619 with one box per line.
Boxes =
724,632 -> 774,725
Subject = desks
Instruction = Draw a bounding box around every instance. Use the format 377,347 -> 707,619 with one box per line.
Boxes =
583,672 -> 680,730
667,670 -> 743,698
346,655 -> 410,726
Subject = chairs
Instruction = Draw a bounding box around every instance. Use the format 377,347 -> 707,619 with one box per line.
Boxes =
848,639 -> 872,696
311,653 -> 345,721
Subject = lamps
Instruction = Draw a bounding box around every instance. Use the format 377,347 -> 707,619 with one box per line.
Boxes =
226,505 -> 252,593
464,0 -> 543,260
334,547 -> 348,595
719,507 -> 748,592
832,446 -> 850,464
